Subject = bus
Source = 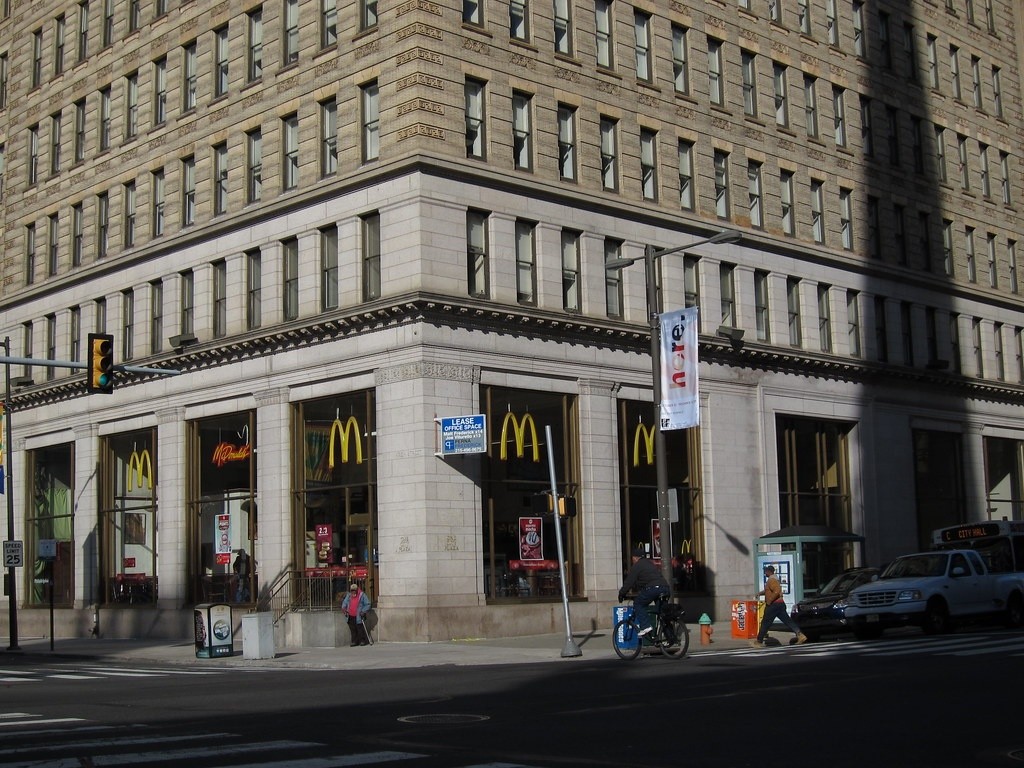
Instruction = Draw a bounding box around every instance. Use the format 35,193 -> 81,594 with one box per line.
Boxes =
933,520 -> 1024,572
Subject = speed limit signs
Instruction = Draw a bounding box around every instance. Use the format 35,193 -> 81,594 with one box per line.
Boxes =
3,541 -> 24,568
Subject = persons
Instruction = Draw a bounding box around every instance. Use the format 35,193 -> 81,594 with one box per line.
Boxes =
618,549 -> 670,646
233,549 -> 250,601
750,566 -> 807,648
342,584 -> 370,647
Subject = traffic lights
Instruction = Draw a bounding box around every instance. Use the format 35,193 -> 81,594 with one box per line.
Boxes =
530,494 -> 552,515
88,332 -> 115,393
558,497 -> 577,517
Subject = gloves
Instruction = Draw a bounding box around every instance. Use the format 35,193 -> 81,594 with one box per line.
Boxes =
618,595 -> 625,603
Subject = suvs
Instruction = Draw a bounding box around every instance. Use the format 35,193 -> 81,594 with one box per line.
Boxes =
790,565 -> 886,643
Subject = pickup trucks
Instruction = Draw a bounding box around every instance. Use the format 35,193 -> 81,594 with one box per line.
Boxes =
842,549 -> 1024,641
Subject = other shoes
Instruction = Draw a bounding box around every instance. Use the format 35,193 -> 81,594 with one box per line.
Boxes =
350,642 -> 357,646
637,624 -> 653,636
654,640 -> 670,646
750,640 -> 764,648
361,641 -> 366,646
795,631 -> 807,644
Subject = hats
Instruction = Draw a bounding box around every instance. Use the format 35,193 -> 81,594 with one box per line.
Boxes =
632,549 -> 644,557
763,565 -> 776,572
350,584 -> 358,591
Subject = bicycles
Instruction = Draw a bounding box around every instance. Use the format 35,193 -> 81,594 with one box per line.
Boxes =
612,591 -> 690,661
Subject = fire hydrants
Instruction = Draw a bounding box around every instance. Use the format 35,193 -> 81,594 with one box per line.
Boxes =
698,613 -> 714,644
